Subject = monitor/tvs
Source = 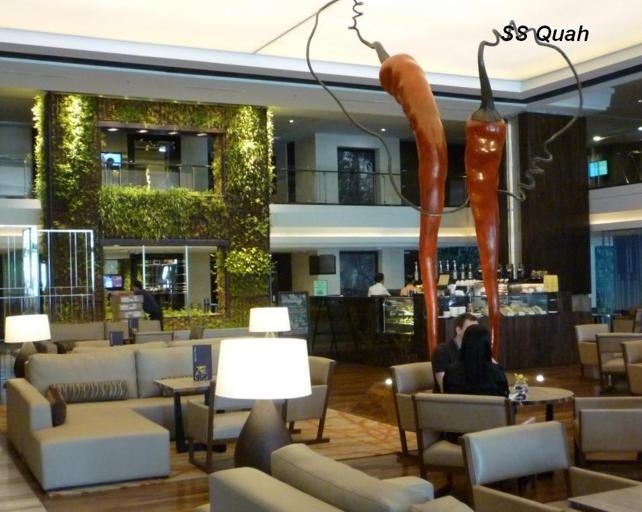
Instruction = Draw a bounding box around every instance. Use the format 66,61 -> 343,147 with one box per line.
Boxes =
588,160 -> 609,177
103,274 -> 124,289
101,152 -> 122,168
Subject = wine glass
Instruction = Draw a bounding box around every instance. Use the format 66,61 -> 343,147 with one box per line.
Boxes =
512,381 -> 528,400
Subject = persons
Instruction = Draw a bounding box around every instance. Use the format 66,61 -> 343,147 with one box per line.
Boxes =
440,323 -> 513,446
367,272 -> 390,297
127,279 -> 162,322
399,274 -> 417,296
432,312 -> 499,441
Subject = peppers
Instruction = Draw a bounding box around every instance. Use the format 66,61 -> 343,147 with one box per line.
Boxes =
464,106 -> 507,365
379,51 -> 449,358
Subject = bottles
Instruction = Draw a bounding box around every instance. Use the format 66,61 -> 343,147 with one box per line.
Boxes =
413,260 -> 547,285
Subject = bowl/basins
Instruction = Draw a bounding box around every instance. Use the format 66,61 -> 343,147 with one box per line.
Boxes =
443,306 -> 466,318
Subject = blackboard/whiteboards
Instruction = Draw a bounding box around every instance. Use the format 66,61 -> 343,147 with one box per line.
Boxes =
278,291 -> 310,337
